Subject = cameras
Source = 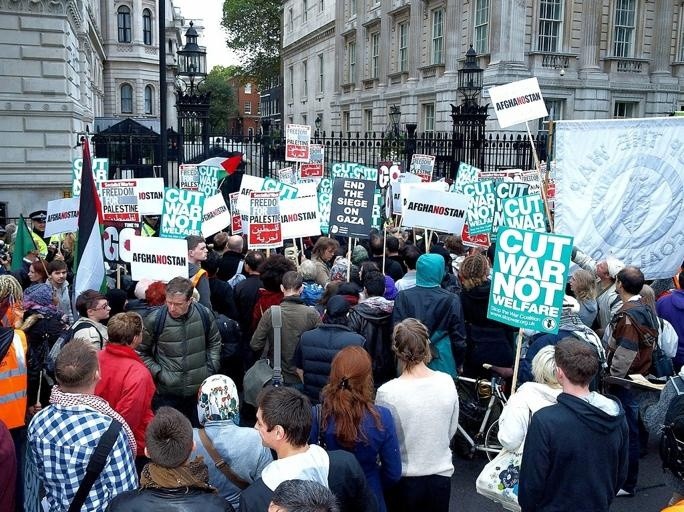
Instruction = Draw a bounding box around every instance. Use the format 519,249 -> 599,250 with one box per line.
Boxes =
0,243 -> 8,260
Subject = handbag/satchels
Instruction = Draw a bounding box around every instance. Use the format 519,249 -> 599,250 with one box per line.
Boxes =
643,349 -> 676,391
226,274 -> 247,290
475,436 -> 530,511
242,358 -> 284,409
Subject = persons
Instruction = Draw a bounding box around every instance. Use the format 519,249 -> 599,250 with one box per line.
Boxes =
0,209 -> 684,512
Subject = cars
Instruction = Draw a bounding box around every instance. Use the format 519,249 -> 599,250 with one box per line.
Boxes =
208,136 -> 251,171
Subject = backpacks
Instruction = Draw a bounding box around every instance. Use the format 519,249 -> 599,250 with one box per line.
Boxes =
43,318 -> 103,385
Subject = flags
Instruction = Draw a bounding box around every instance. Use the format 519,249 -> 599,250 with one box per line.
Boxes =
68,141 -> 109,300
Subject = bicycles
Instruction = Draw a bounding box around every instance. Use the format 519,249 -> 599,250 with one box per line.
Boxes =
448,363 -> 512,464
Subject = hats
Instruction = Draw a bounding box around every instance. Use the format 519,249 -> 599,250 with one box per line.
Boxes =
458,254 -> 490,279
29,210 -> 47,223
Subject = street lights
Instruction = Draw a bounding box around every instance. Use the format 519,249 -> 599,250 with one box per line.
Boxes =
173,19 -> 213,164
450,42 -> 490,177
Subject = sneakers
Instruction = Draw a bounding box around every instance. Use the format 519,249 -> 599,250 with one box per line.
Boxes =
616,486 -> 636,498
